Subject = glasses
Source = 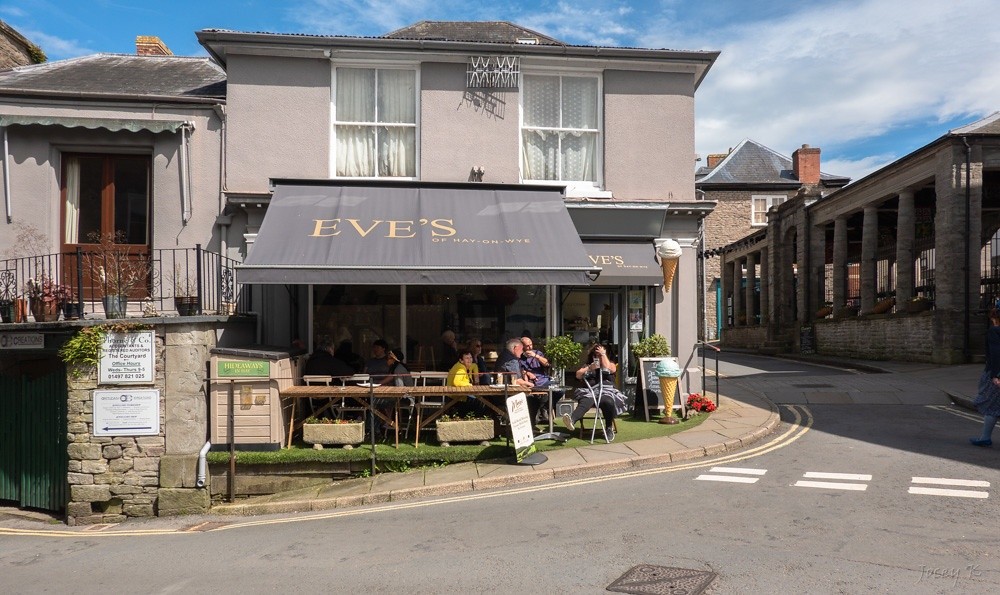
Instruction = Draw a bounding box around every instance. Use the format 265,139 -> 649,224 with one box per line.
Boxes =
476,345 -> 482,348
386,357 -> 394,361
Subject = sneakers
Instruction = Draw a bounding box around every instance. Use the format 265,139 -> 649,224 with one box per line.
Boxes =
562,413 -> 575,431
969,438 -> 993,445
532,426 -> 542,433
605,428 -> 615,442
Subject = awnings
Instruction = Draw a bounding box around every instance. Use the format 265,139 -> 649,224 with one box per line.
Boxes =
235,178 -> 595,285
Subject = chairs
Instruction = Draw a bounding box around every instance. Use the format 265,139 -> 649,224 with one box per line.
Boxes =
303,371 -> 449,439
579,401 -> 617,440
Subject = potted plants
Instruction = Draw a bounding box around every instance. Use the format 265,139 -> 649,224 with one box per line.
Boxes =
0,215 -> 198,323
303,415 -> 366,450
543,333 -> 584,418
435,411 -> 494,447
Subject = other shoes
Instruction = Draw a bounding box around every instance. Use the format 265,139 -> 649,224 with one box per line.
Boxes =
540,408 -> 548,419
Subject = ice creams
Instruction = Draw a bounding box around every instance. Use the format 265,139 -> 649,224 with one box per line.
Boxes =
658,238 -> 682,292
656,360 -> 681,416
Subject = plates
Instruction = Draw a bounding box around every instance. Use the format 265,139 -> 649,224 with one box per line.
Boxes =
357,382 -> 381,388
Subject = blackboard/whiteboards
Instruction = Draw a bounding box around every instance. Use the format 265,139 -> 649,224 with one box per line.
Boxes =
635,356 -> 685,410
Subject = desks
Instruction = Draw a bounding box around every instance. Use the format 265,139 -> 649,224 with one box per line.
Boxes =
279,385 -> 574,449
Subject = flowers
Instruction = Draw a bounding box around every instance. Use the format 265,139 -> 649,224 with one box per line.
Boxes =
686,393 -> 717,412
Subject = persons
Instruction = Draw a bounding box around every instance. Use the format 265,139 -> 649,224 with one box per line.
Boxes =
334,339 -> 365,420
378,349 -> 414,437
969,307 -> 1000,447
445,351 -> 489,417
518,336 -> 564,422
563,344 -> 617,441
305,340 -> 358,420
467,338 -> 491,385
361,339 -> 396,443
494,337 -> 543,444
439,330 -> 459,364
